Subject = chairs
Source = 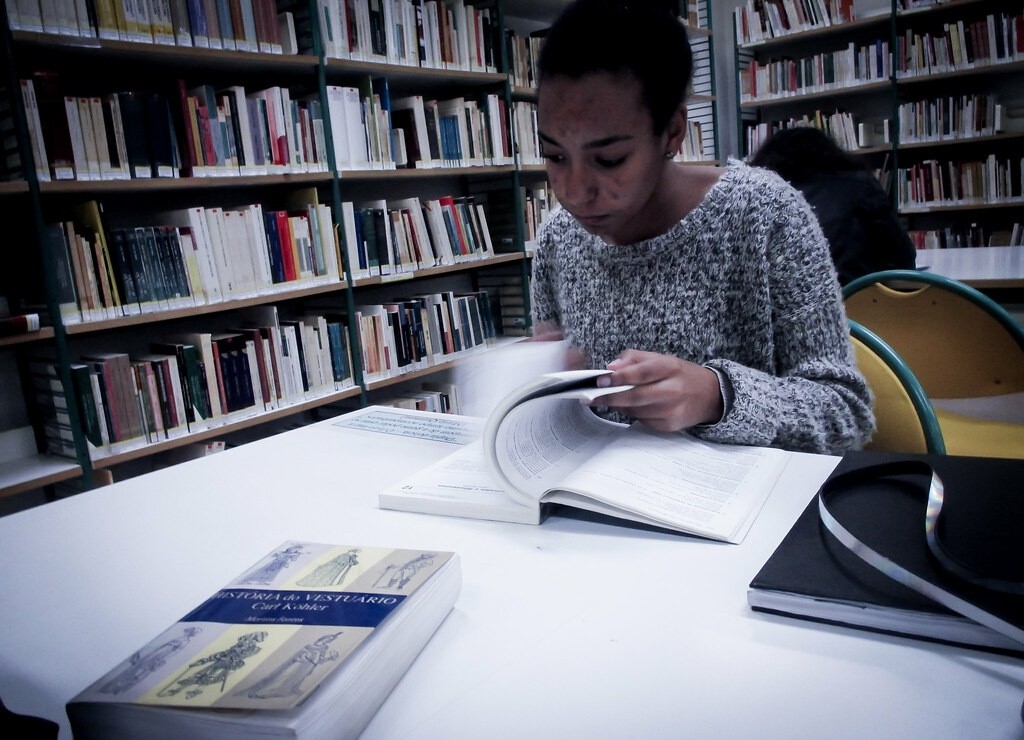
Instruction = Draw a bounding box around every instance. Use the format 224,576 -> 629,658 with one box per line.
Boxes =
842,269 -> 1024,397
848,319 -> 947,454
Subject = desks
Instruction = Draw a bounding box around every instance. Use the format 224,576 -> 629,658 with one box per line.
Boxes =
886,246 -> 1024,288
0,404 -> 1024,740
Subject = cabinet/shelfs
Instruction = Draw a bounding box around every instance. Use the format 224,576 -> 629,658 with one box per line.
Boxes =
0,0 -> 1024,499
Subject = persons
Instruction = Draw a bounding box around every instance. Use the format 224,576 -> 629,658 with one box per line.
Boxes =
532,1 -> 881,460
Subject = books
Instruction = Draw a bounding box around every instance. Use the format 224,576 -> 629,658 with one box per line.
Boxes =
67,539 -> 463,739
380,367 -> 795,542
0,1 -> 1024,456
747,450 -> 1024,654
